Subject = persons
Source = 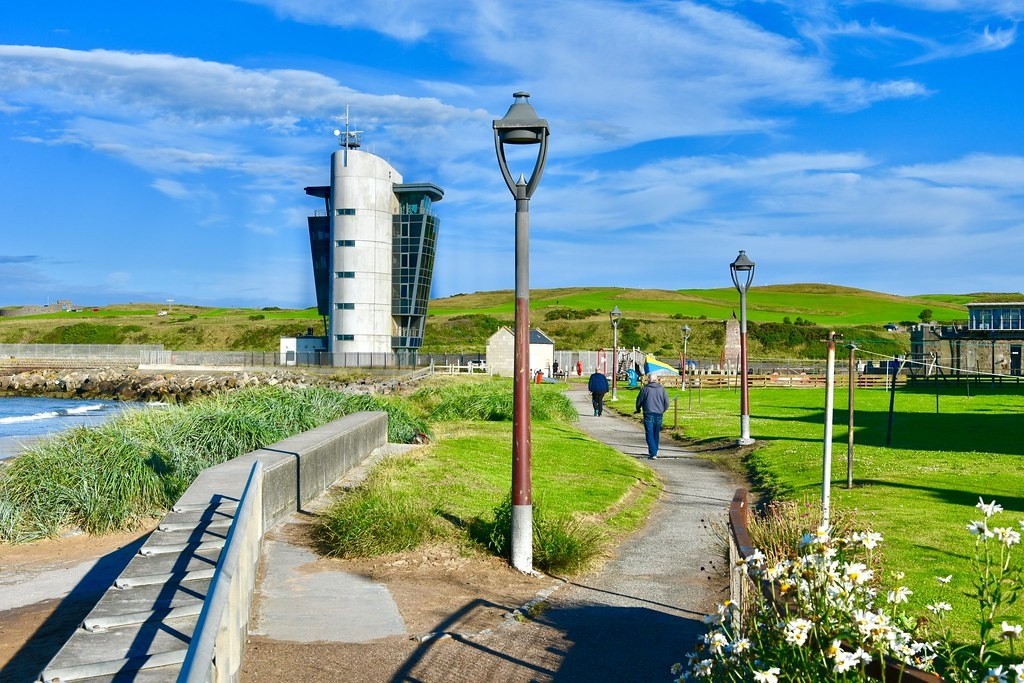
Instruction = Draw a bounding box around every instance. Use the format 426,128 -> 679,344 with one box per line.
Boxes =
588,367 -> 610,416
553,360 -> 559,377
636,373 -> 670,460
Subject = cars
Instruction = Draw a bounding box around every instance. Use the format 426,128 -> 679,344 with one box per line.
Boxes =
882,324 -> 899,331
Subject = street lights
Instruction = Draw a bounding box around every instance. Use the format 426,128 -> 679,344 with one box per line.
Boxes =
166,299 -> 174,313
681,324 -> 691,391
609,304 -> 623,402
491,89 -> 552,577
729,250 -> 757,445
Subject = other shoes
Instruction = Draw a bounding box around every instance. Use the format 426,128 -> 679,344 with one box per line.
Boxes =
599,414 -> 602,416
594,409 -> 598,416
648,456 -> 657,460
